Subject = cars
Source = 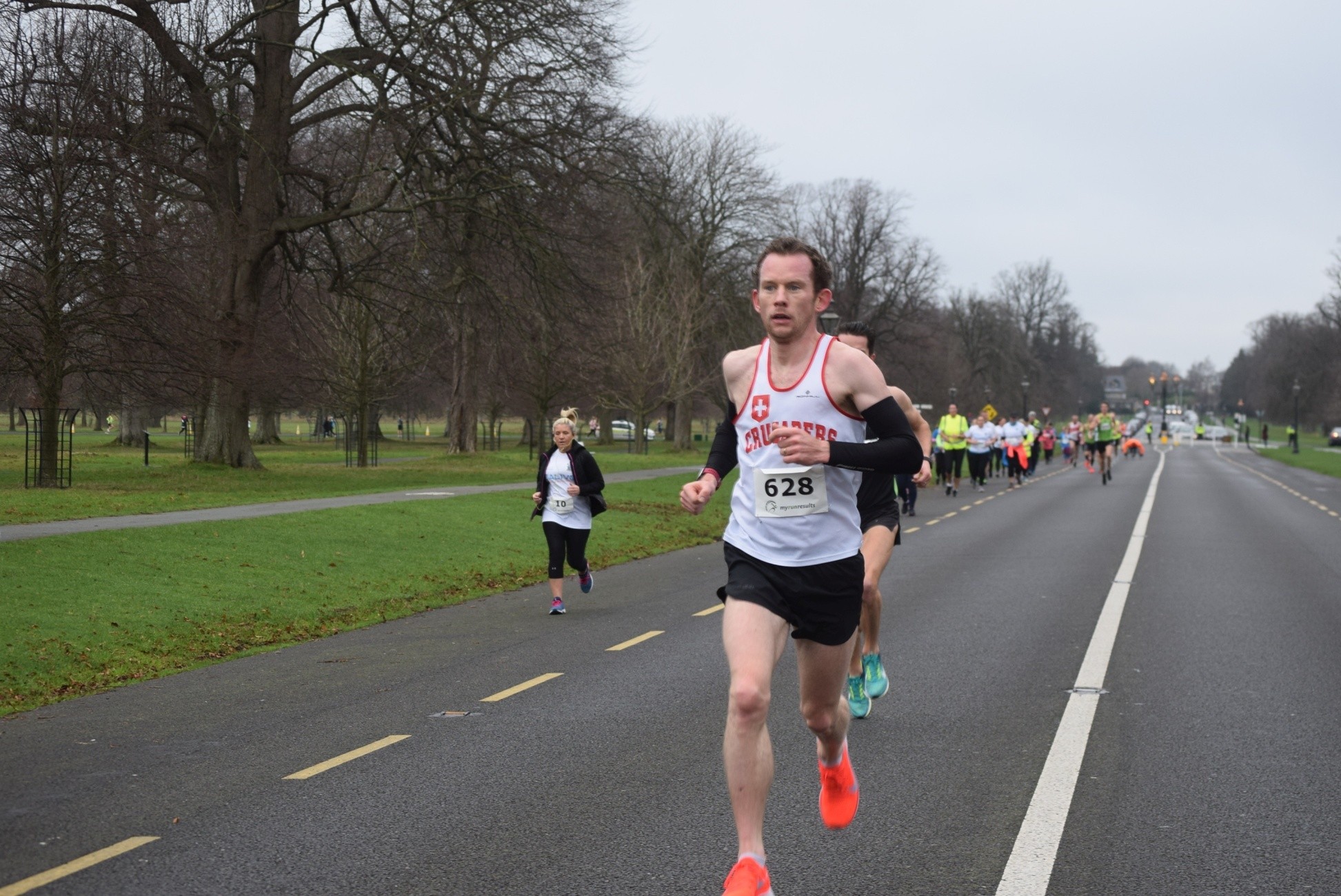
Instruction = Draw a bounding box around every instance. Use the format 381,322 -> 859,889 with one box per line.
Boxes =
595,419 -> 656,441
1329,426 -> 1341,448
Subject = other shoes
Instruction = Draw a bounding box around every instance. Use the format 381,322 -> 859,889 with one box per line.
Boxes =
971,457 -> 1113,493
901,502 -> 909,513
937,478 -> 939,484
908,507 -> 915,515
953,490 -> 957,496
945,487 -> 952,495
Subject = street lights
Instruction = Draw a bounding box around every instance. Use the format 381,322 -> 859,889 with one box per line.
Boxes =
1291,381 -> 1303,453
1149,372 -> 1180,438
1020,379 -> 1030,421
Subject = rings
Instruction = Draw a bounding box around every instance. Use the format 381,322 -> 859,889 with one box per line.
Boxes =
924,483 -> 926,484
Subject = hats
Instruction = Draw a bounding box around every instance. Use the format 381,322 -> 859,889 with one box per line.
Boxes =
1010,412 -> 1017,418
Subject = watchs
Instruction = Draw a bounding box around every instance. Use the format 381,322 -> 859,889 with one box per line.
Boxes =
923,457 -> 933,469
696,468 -> 722,491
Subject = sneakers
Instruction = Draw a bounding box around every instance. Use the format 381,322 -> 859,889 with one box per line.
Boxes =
720,856 -> 774,896
549,597 -> 566,615
578,563 -> 593,593
819,736 -> 860,830
862,652 -> 889,700
848,661 -> 872,718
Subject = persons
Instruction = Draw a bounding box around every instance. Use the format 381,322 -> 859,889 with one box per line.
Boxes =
1112,419 -> 1144,457
1286,426 -> 1295,447
657,417 -> 663,433
105,413 -> 113,434
588,417 -> 597,437
179,415 -> 188,436
1262,424 -> 1268,446
1245,426 -> 1249,447
530,407 -> 607,615
677,238 -> 923,896
323,417 -> 336,438
1146,421 -> 1152,443
397,418 -> 403,437
895,476 -> 917,516
931,425 -> 947,487
1196,423 -> 1205,439
937,401 -> 1127,496
835,323 -> 932,720
